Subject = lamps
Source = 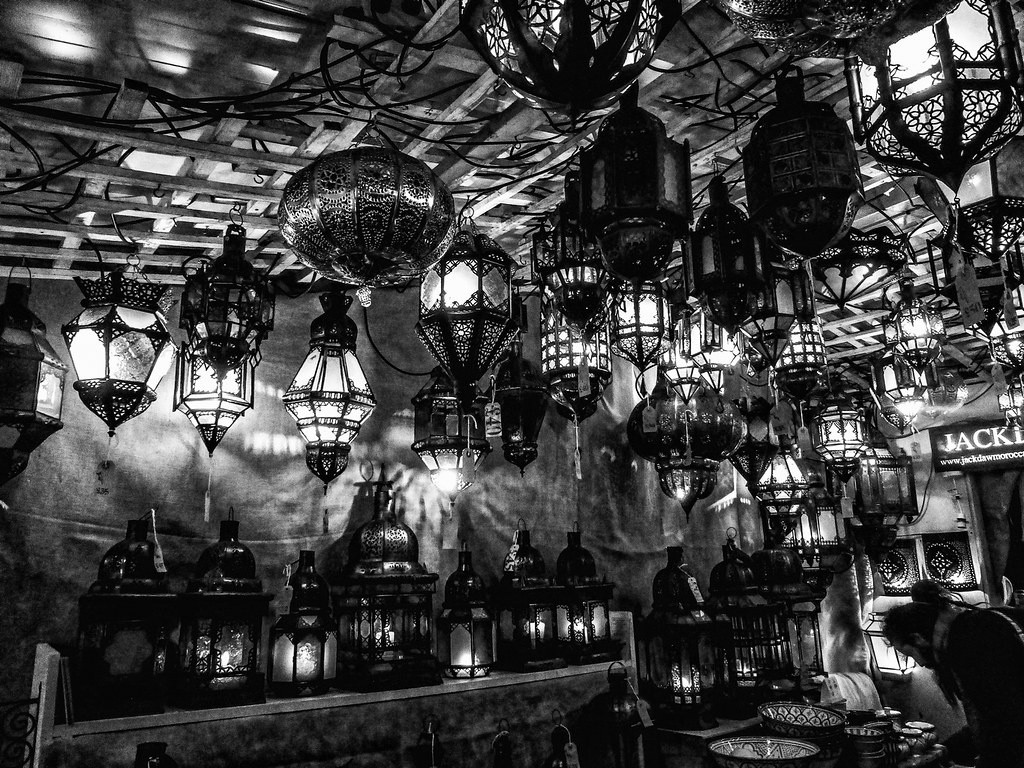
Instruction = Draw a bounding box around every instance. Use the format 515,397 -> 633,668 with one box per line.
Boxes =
0,0 -> 1024,732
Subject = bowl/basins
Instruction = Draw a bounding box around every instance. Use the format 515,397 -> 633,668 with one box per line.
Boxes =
708,701 -> 897,768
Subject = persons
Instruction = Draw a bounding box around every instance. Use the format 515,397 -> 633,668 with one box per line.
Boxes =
880,583 -> 1024,768
